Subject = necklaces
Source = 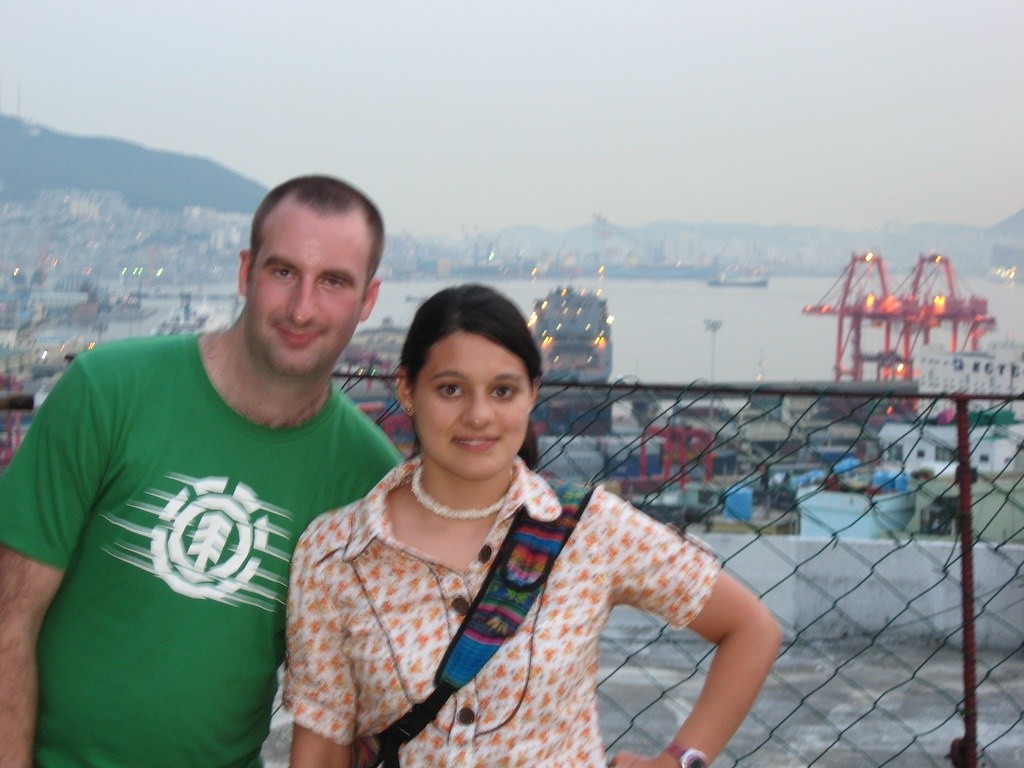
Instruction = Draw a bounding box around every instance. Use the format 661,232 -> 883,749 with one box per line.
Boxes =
412,465 -> 513,519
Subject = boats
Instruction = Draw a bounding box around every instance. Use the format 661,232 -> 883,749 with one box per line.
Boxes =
706,277 -> 770,289
532,288 -> 612,385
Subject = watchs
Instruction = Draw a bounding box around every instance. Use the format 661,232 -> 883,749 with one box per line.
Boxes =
665,744 -> 708,768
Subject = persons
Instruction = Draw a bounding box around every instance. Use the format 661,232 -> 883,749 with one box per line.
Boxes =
1,175 -> 405,768
284,283 -> 780,768
